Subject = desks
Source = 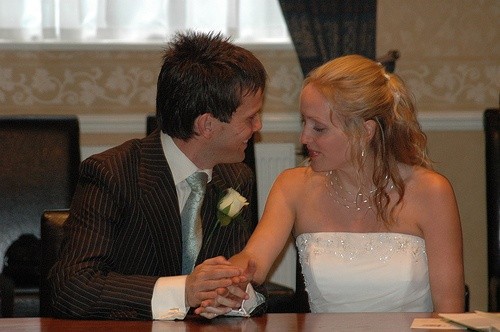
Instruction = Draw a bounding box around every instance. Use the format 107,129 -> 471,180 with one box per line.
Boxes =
0,312 -> 477,332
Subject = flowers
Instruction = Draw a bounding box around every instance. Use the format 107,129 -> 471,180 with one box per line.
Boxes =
202,187 -> 249,250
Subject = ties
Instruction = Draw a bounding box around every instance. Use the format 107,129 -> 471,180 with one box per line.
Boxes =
179,172 -> 208,276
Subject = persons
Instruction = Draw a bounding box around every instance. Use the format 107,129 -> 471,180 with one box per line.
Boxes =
47,31 -> 270,322
190,56 -> 465,316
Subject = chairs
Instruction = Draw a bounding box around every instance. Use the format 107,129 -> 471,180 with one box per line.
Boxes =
0,114 -> 81,317
146,116 -> 295,313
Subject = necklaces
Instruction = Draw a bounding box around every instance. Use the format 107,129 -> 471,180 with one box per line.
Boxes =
323,166 -> 395,211
